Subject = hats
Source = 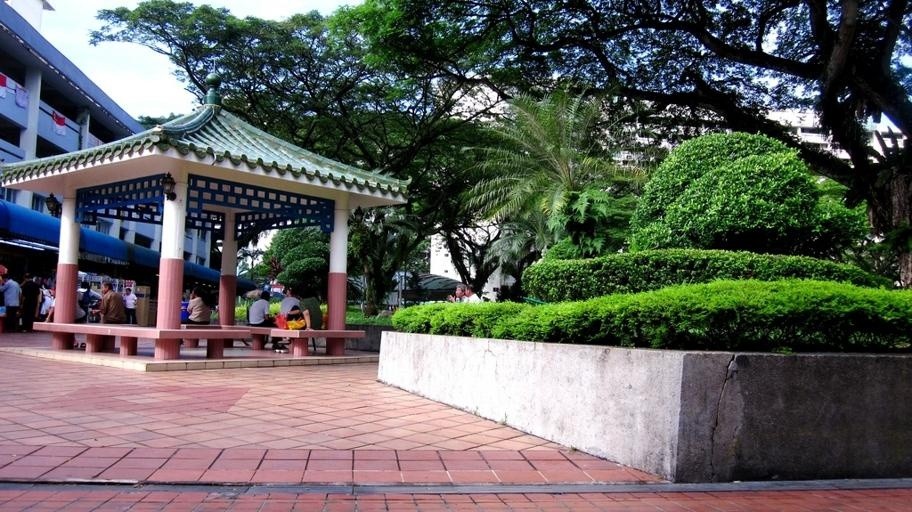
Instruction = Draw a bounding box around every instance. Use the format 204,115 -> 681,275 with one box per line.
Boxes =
260,291 -> 272,298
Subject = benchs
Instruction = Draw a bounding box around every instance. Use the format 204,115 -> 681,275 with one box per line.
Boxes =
31,319 -> 367,358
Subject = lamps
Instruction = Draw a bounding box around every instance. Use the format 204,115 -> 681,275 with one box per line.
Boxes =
46,192 -> 62,216
161,171 -> 177,202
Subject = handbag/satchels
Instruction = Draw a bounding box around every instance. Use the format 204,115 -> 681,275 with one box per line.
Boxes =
274,315 -> 306,329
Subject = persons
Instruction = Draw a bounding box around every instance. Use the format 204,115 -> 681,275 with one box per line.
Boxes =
46,285 -> 86,348
180,288 -> 219,345
248,286 -> 323,353
123,288 -> 138,324
76,281 -> 102,322
465,284 -> 481,302
100,282 -> 126,323
0,273 -> 56,333
447,282 -> 466,303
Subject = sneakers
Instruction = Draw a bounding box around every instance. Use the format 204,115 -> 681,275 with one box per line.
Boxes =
272,344 -> 288,352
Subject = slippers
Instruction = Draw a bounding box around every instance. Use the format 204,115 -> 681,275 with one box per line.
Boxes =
74,341 -> 86,348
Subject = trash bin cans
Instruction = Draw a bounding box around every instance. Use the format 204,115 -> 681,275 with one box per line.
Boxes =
148,300 -> 157,326
182,302 -> 189,324
